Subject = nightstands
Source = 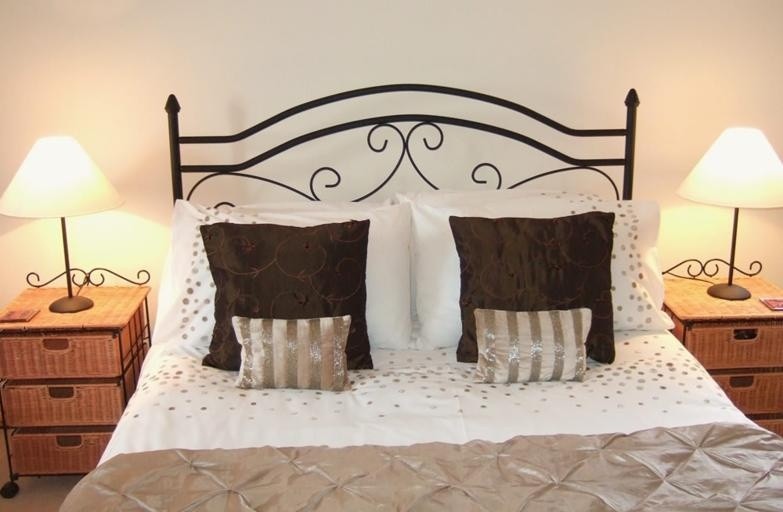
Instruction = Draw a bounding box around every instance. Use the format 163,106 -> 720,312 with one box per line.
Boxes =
660,275 -> 782,437
0,285 -> 153,498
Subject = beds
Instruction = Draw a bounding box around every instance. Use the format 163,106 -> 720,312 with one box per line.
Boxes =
55,83 -> 783,511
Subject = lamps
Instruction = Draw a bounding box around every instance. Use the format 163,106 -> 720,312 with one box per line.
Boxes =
0,135 -> 125,314
676,126 -> 783,301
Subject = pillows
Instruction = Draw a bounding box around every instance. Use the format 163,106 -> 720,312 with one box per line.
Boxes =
155,196 -> 415,349
449,209 -> 616,364
408,193 -> 675,349
473,308 -> 597,383
232,314 -> 353,391
201,219 -> 374,373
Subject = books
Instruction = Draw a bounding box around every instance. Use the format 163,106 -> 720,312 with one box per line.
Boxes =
759,297 -> 783,311
0,309 -> 40,323
